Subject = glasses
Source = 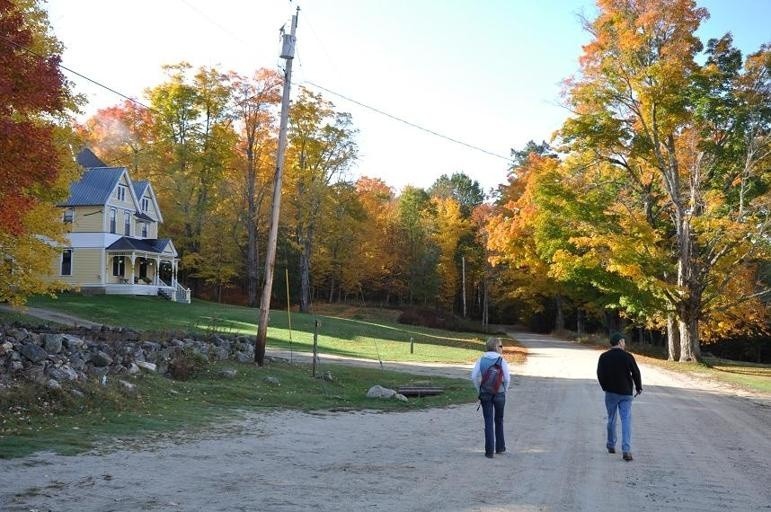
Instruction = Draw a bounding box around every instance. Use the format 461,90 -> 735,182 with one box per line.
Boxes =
499,345 -> 503,348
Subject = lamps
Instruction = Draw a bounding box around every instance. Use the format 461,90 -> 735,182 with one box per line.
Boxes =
138,258 -> 154,266
118,259 -> 123,264
164,264 -> 171,272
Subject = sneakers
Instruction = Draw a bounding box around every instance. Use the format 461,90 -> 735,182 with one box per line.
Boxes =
485,448 -> 506,458
607,447 -> 634,461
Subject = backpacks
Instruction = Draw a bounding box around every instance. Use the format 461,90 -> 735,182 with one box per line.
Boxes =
479,355 -> 504,395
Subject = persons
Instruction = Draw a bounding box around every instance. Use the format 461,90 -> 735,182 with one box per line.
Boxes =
595,331 -> 644,461
470,338 -> 511,458
